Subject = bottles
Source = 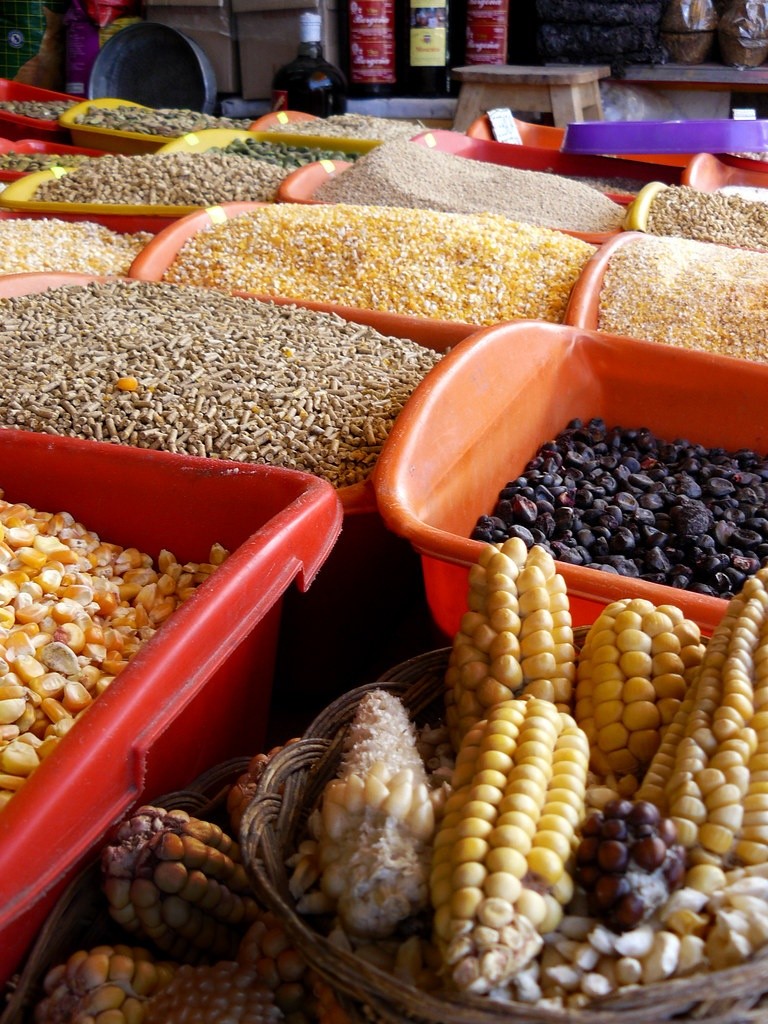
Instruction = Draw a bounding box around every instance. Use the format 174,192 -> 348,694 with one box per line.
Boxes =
401,0 -> 455,99
269,11 -> 348,119
461,0 -> 525,67
344,0 -> 401,99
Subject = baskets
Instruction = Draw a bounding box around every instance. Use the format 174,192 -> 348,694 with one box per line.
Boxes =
239,623 -> 768,1024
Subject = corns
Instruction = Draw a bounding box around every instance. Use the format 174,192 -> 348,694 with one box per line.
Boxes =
309,534 -> 768,996
26,734 -> 355,1024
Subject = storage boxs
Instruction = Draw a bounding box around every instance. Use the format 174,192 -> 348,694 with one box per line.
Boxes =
0,78 -> 768,1024
144,0 -> 239,97
231,0 -> 339,105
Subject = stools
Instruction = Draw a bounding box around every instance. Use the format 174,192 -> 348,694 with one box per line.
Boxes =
446,63 -> 614,131
